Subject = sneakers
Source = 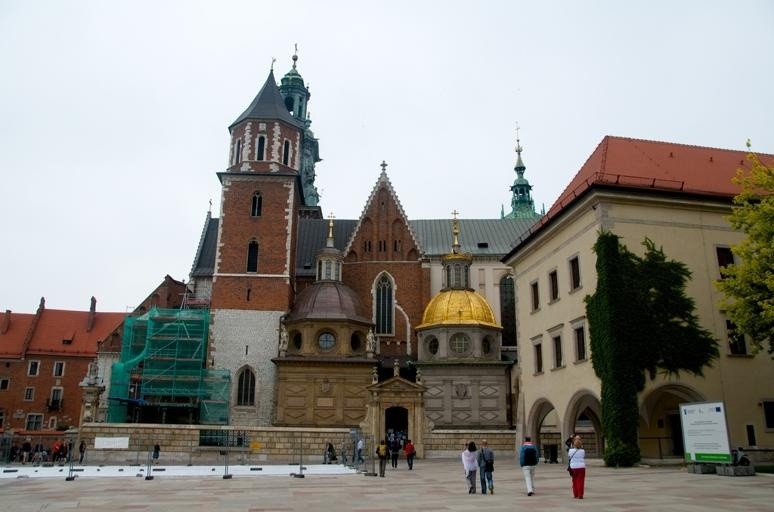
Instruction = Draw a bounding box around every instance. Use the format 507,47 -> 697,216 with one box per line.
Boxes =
469,488 -> 494,494
527,493 -> 533,496
574,496 -> 583,499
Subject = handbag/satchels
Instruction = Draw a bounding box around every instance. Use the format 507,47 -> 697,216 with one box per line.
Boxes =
568,464 -> 572,476
484,462 -> 494,472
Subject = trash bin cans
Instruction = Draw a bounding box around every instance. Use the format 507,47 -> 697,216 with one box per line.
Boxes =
543,444 -> 558,464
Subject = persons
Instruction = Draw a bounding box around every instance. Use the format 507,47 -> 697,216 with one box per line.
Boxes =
151,442 -> 160,464
357,439 -> 364,463
328,443 -> 337,464
375,426 -> 415,477
477,440 -> 494,494
461,442 -> 479,494
567,440 -> 586,499
9,437 -> 86,465
519,436 -> 539,496
565,434 -> 581,448
